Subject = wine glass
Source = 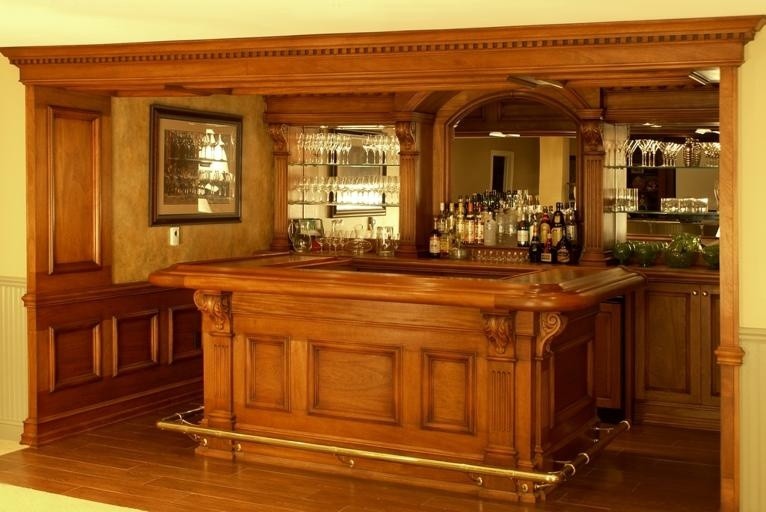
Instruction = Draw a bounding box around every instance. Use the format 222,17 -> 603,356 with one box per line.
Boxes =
295,175 -> 400,206
294,132 -> 353,166
163,128 -> 235,204
314,220 -> 376,257
614,135 -> 719,168
361,133 -> 400,165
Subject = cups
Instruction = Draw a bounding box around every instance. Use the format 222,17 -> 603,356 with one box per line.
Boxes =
610,187 -> 638,212
288,222 -> 312,253
661,197 -> 709,214
377,226 -> 400,257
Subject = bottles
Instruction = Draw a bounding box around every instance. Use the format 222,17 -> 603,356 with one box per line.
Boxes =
428,188 -> 578,266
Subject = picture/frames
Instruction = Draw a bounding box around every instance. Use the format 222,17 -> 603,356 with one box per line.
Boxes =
149,104 -> 243,226
330,128 -> 388,217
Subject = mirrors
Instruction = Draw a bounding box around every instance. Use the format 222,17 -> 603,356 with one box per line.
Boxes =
615,120 -> 719,244
448,135 -> 575,210
288,126 -> 400,242
